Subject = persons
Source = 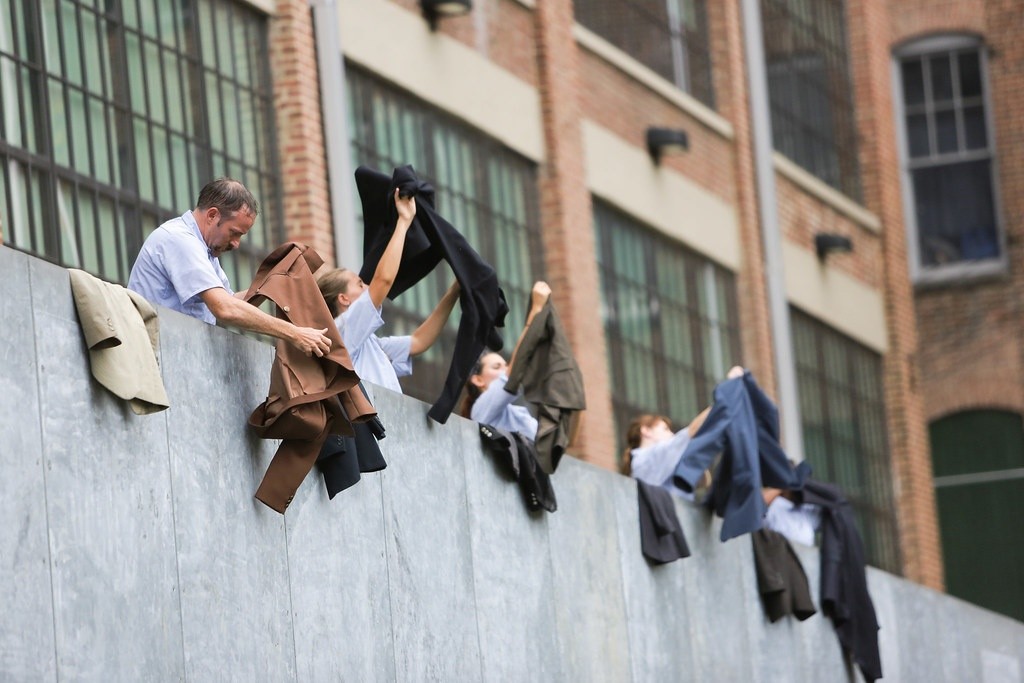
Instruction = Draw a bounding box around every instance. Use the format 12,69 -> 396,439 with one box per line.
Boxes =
755,459 -> 825,546
466,282 -> 552,441
126,176 -> 332,359
317,188 -> 459,395
623,365 -> 744,503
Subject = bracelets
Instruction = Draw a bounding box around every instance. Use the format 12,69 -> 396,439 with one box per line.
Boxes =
524,323 -> 532,328
535,308 -> 541,313
